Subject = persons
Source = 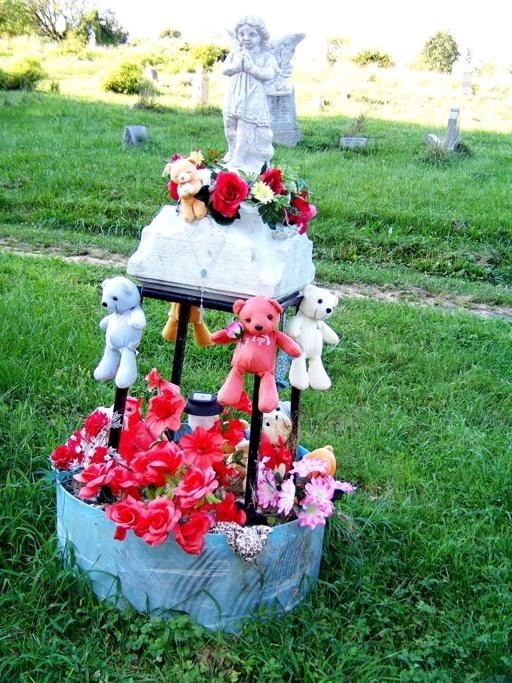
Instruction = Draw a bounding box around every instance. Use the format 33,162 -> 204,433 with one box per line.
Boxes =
218,13 -> 307,169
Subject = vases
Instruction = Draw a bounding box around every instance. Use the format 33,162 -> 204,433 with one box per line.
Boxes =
49,480 -> 331,636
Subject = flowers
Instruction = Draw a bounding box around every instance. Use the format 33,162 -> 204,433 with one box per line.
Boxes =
43,368 -> 357,560
160,142 -> 319,241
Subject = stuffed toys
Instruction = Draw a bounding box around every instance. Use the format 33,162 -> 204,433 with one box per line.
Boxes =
159,157 -> 208,222
163,300 -> 215,349
208,295 -> 302,415
286,282 -> 341,392
183,391 -> 337,566
93,275 -> 148,388
